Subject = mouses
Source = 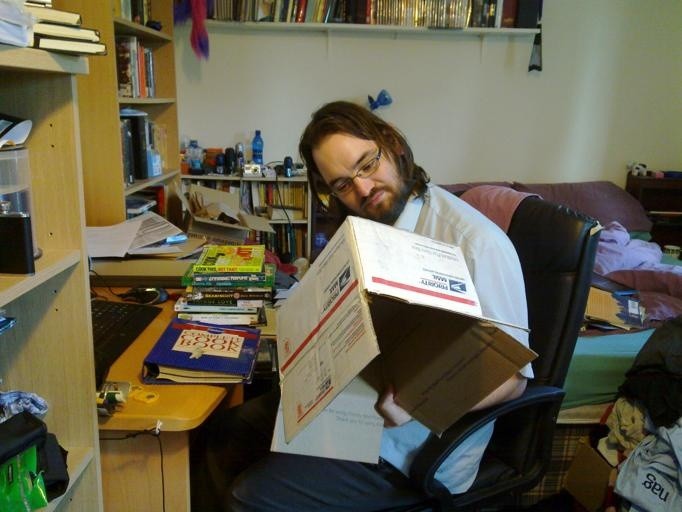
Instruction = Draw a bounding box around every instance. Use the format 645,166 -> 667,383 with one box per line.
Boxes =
122,285 -> 169,305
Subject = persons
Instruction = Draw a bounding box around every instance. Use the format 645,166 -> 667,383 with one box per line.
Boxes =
195,101 -> 535,511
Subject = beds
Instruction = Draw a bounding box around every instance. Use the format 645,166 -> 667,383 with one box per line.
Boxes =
440,180 -> 682,426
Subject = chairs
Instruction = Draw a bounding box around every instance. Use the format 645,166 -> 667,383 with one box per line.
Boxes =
414,179 -> 602,510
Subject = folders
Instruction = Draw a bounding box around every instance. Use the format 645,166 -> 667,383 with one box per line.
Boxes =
142,318 -> 262,385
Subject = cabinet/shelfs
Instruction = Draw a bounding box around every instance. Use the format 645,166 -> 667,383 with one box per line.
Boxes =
175,0 -> 544,38
60,0 -> 183,223
181,165 -> 316,269
0,43 -> 104,511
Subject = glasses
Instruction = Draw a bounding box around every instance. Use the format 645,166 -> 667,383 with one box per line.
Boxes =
330,143 -> 382,199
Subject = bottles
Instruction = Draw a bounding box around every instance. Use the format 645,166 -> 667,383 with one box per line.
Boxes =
252,129 -> 264,165
215,143 -> 245,176
184,140 -> 207,176
312,232 -> 328,267
283,156 -> 292,178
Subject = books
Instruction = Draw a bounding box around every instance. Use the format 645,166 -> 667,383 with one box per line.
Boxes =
207,0 -> 538,28
582,271 -> 651,331
28,5 -> 83,27
32,22 -> 101,44
115,1 -> 168,245
141,178 -> 309,386
34,36 -> 108,56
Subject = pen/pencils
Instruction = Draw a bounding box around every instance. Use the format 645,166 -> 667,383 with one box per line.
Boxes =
95,391 -> 125,416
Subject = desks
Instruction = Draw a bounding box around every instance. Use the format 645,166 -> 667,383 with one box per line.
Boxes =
90,259 -> 246,512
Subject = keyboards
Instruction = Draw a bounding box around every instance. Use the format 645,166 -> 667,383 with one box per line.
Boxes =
91,299 -> 163,380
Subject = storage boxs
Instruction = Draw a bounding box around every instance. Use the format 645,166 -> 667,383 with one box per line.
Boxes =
275,215 -> 538,442
